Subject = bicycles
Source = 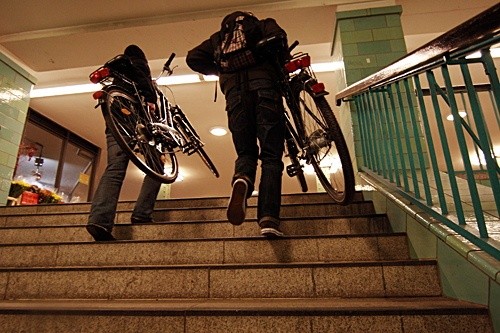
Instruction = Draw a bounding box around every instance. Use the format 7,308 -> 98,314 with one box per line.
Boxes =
89,52 -> 220,184
275,39 -> 356,207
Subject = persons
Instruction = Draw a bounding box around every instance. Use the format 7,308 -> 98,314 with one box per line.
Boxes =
84,44 -> 166,241
186,11 -> 294,236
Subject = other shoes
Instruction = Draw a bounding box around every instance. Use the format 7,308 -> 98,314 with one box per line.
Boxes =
131,218 -> 156,224
226,178 -> 249,225
260,221 -> 283,236
86,224 -> 116,241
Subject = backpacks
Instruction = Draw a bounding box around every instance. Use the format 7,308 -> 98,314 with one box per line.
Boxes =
218,15 -> 259,73
